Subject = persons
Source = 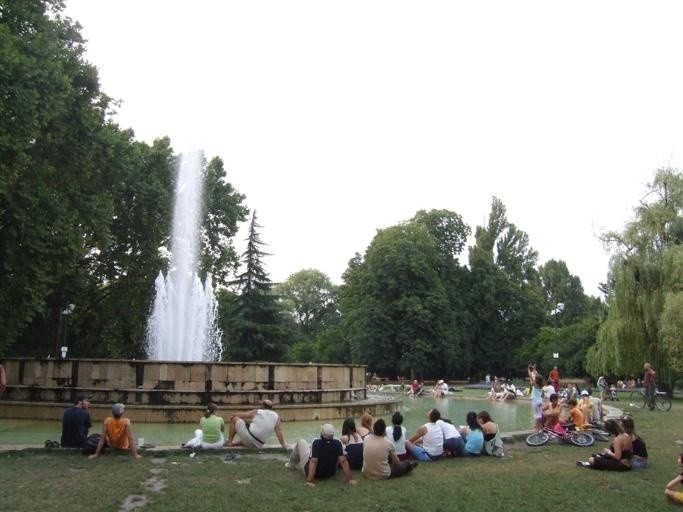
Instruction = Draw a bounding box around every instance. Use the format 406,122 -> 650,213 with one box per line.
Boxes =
366,370 -> 377,384
285,408 -> 505,487
194,402 -> 225,450
61,391 -> 92,448
467,373 -> 530,401
527,364 -> 648,472
88,403 -> 142,459
401,379 -> 449,399
224,400 -> 288,448
665,453 -> 683,505
642,362 -> 656,410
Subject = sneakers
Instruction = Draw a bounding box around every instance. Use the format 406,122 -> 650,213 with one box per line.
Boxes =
576,460 -> 590,468
140,442 -> 155,449
44,439 -> 59,448
224,451 -> 241,460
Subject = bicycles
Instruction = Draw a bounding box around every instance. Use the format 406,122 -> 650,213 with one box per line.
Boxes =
629,381 -> 672,412
586,418 -> 609,442
525,421 -> 594,447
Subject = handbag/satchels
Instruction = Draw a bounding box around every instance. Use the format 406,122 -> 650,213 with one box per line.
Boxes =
83,433 -> 107,455
484,424 -> 505,457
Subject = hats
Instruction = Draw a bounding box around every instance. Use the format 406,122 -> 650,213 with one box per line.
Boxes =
321,423 -> 335,440
76,393 -> 95,400
580,390 -> 589,396
112,403 -> 125,414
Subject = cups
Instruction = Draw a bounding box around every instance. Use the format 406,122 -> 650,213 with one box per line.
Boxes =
137,437 -> 145,446
